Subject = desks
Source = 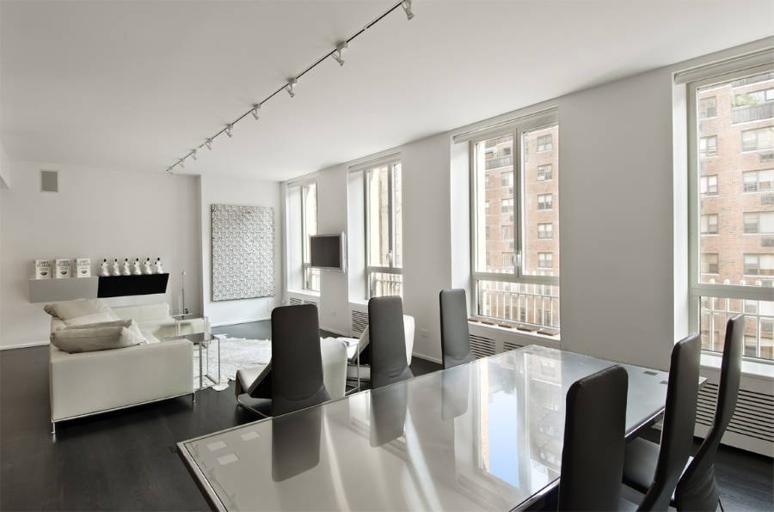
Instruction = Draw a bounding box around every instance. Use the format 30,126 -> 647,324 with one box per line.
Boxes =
172,344 -> 708,511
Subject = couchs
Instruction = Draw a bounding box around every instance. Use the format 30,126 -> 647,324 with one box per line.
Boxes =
47,301 -> 198,443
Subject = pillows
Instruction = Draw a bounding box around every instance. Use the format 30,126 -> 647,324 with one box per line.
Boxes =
42,299 -> 147,353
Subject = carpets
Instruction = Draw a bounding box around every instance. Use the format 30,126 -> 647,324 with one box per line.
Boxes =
187,333 -> 276,389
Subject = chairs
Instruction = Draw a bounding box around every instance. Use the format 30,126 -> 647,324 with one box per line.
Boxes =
366,293 -> 416,388
269,300 -> 341,416
438,287 -> 478,369
625,313 -> 748,512
618,330 -> 703,509
551,361 -> 631,512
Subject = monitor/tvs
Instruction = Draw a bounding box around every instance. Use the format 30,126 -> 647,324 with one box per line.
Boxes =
309,233 -> 346,273
